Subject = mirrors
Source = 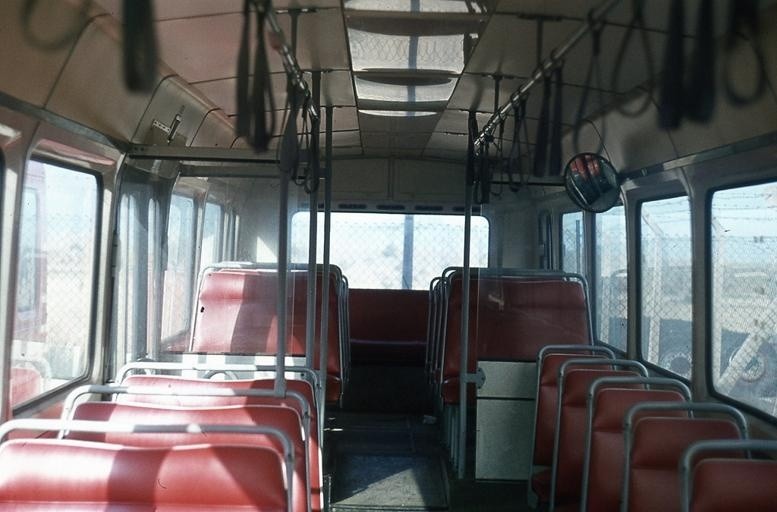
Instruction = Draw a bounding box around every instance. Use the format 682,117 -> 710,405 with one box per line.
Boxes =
565,152 -> 621,213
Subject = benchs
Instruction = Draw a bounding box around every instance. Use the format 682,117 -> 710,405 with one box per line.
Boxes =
342,287 -> 430,413
424,265 -> 596,476
189,262 -> 350,404
2,359 -> 326,512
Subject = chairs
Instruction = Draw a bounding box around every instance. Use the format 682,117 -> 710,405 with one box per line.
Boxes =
531,345 -> 773,507
